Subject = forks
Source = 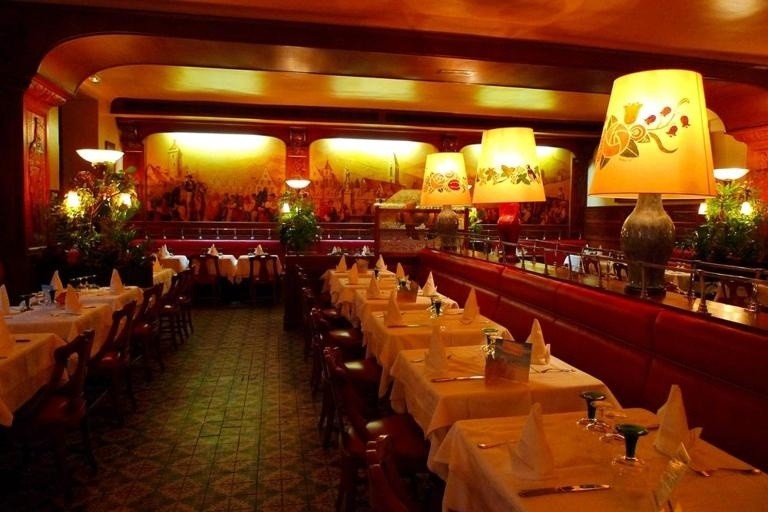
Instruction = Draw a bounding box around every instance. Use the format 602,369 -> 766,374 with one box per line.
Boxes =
532,367 -> 574,373
689,466 -> 760,477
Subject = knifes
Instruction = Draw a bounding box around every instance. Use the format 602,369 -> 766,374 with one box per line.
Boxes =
387,325 -> 421,328
519,485 -> 610,497
431,375 -> 484,382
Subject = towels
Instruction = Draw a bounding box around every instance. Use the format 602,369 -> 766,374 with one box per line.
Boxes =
252,244 -> 264,255
207,244 -> 218,256
336,255 -> 347,272
376,254 -> 387,271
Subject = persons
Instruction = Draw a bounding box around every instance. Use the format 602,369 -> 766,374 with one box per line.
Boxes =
339,204 -> 352,222
324,201 -> 337,223
146,175 -> 277,222
521,186 -> 568,225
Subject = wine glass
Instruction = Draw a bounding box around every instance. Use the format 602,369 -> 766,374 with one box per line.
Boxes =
71,275 -> 95,289
48,290 -> 58,302
427,295 -> 443,320
480,327 -> 502,358
32,293 -> 42,306
394,277 -> 406,291
576,392 -> 606,425
21,294 -> 34,311
611,425 -> 647,472
586,401 -> 612,433
600,413 -> 624,445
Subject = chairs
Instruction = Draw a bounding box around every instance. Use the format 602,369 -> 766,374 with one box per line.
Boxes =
282,263 -> 438,512
241,253 -> 281,309
189,254 -> 228,308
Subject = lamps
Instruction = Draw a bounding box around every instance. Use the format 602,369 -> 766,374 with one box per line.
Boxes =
285,179 -> 311,190
420,152 -> 472,250
472,126 -> 546,263
587,69 -> 718,296
76,149 -> 124,166
713,168 -> 750,182
